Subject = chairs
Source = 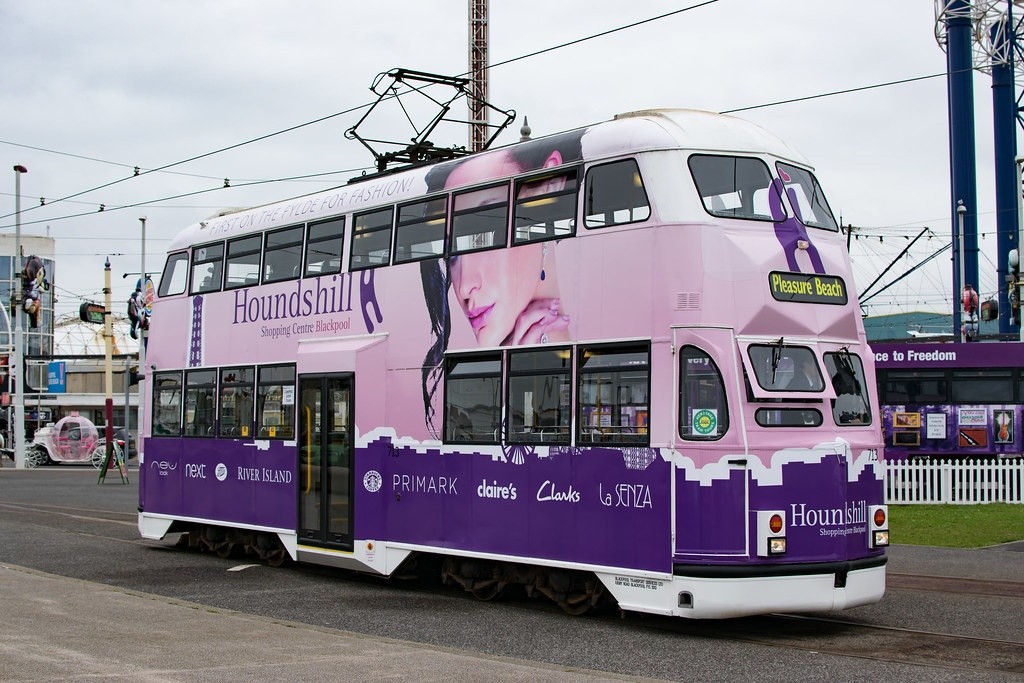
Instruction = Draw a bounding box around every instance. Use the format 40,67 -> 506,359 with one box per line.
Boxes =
453,428 -> 462,441
493,428 -> 499,441
260,426 -> 282,436
231,426 -> 239,434
208,426 -> 214,435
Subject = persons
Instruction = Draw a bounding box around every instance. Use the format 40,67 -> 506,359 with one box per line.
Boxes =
421,128 -> 585,440
781,352 -> 822,425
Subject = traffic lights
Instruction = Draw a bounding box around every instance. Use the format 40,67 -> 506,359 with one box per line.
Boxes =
45,283 -> 50,291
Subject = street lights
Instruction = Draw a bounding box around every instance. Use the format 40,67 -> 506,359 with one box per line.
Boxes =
1015,153 -> 1024,341
138,217 -> 145,433
15,165 -> 28,469
957,206 -> 965,343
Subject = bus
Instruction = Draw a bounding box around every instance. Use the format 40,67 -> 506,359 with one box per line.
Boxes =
137,68 -> 891,620
563,339 -> 1023,499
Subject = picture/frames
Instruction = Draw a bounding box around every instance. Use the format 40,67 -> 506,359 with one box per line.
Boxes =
893,412 -> 920,428
621,414 -> 630,426
992,410 -> 1014,444
893,431 -> 921,446
634,410 -> 647,433
926,412 -> 947,440
590,412 -> 611,433
957,428 -> 987,447
958,408 -> 988,426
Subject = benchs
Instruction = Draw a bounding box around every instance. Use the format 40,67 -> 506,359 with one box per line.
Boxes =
78,437 -> 92,449
199,281 -> 205,291
292,264 -> 323,277
353,260 -> 380,269
527,225 -> 569,239
568,219 -> 619,233
381,249 -> 438,264
244,272 -> 269,284
54,436 -> 71,449
226,277 -> 244,287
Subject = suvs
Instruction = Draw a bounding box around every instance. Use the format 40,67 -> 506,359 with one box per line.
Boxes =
62,425 -> 138,465
